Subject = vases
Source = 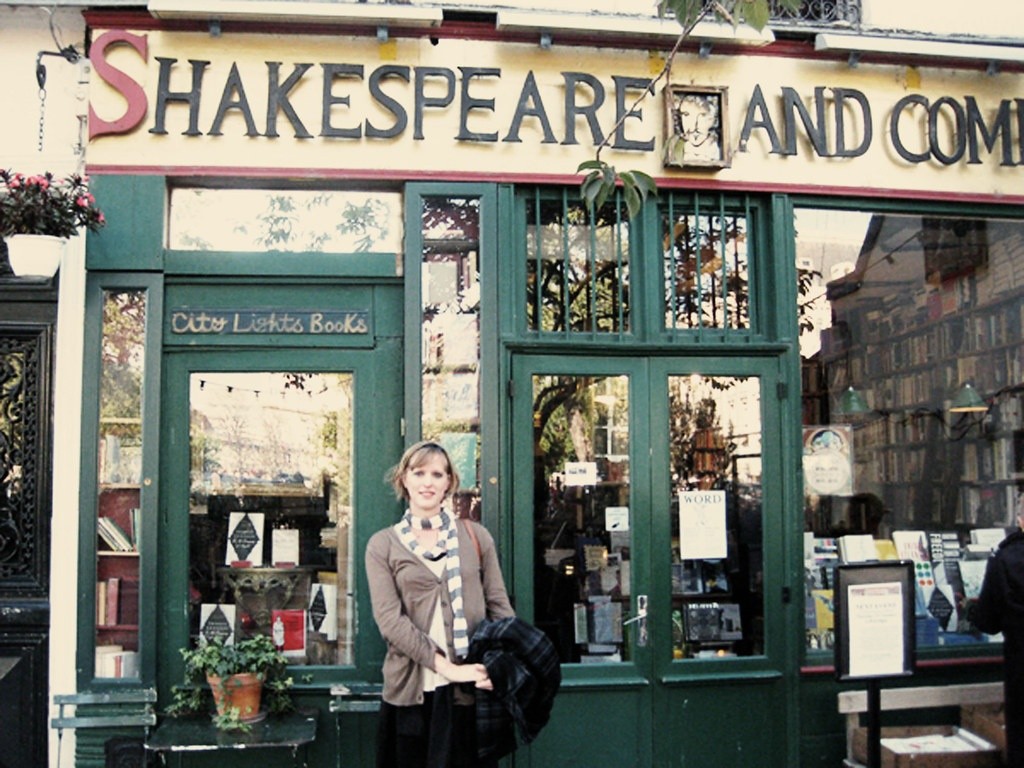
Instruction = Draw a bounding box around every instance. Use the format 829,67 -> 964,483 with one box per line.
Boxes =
3,234 -> 67,277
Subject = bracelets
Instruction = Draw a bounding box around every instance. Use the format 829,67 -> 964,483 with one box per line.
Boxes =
438,660 -> 451,675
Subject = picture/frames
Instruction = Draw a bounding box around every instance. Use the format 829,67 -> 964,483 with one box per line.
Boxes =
683,600 -> 745,644
664,84 -> 731,168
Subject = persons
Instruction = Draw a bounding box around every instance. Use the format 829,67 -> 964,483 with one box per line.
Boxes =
956,485 -> 1024,768
365,440 -> 517,768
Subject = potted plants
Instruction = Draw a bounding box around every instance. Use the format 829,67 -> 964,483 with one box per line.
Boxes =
164,634 -> 295,732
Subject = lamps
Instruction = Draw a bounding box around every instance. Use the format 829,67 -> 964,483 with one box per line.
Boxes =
949,223 -> 990,412
831,269 -> 872,415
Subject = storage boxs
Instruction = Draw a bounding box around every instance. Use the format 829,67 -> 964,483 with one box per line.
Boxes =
960,702 -> 1007,766
852,724 -> 1001,768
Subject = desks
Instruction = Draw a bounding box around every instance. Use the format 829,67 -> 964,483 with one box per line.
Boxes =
216,566 -> 313,634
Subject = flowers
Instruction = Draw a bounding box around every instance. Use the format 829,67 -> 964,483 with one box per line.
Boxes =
0,169 -> 106,239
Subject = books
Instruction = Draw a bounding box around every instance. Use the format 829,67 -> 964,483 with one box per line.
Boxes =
94,644 -> 135,678
99,435 -> 121,484
96,578 -> 121,626
95,504 -> 144,553
568,273 -> 1024,652
190,404 -> 353,665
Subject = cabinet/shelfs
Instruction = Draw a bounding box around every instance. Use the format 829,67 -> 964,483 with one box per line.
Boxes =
94,484 -> 139,677
802,250 -> 1023,533
